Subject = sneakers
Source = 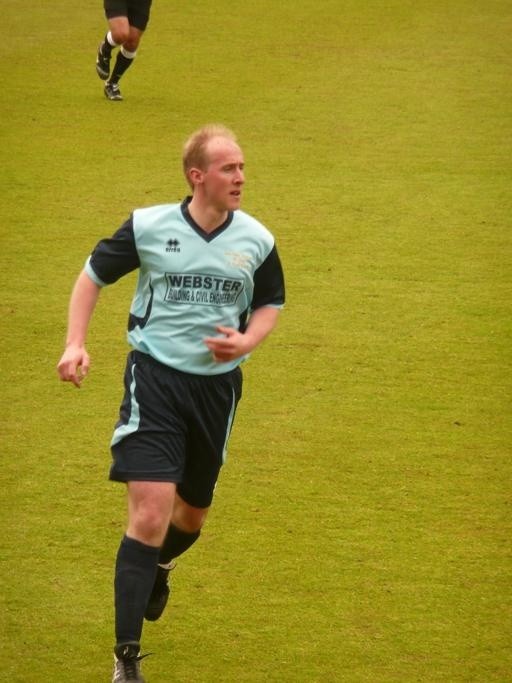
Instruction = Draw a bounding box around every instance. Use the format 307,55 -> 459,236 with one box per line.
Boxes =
144,559 -> 178,621
110,640 -> 144,683
104,79 -> 123,100
95,40 -> 111,80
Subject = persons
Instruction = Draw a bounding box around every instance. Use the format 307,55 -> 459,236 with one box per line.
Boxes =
93,0 -> 155,102
54,120 -> 288,680
